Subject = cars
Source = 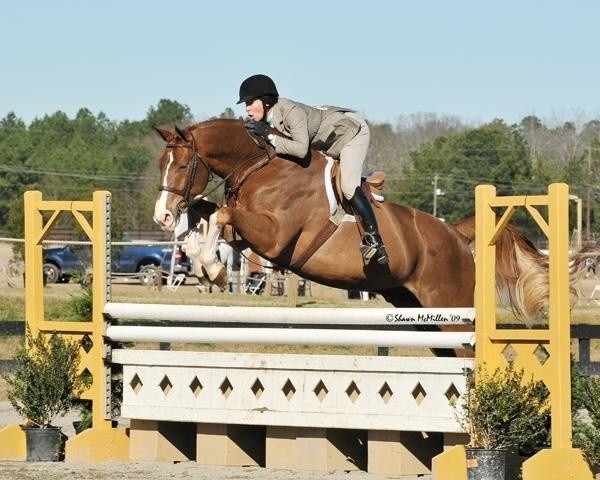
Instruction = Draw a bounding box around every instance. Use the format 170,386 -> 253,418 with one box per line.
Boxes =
43,232 -> 190,286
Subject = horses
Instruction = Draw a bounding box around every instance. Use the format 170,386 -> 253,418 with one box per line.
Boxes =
148,114 -> 600,356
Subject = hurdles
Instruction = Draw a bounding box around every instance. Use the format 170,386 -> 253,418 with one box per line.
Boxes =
0,183 -> 594,480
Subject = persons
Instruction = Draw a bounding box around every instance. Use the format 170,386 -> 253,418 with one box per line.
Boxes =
585,258 -> 598,280
236,74 -> 389,267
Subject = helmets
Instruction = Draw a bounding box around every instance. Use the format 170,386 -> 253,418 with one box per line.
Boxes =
235,74 -> 279,109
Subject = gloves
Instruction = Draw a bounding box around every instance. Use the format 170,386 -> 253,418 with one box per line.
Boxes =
244,119 -> 269,144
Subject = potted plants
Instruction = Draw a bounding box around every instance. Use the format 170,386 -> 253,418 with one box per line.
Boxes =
0,320 -> 92,462
448,361 -> 551,479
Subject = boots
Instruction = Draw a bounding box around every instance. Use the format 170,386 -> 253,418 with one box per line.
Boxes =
348,187 -> 389,265
